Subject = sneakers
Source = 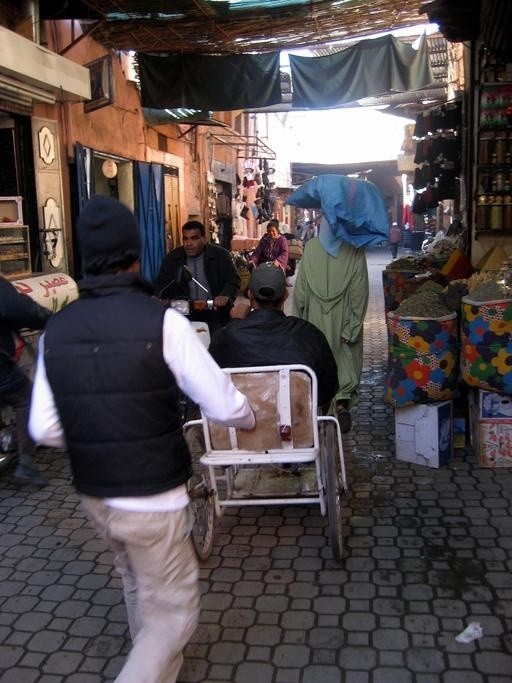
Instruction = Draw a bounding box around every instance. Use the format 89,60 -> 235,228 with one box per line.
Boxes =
338,408 -> 351,434
14,463 -> 47,486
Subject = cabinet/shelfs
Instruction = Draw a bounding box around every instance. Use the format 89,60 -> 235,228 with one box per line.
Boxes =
470,37 -> 512,271
0,226 -> 32,281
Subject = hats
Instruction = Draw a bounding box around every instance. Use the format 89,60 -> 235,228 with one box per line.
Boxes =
79,194 -> 141,261
249,262 -> 285,301
392,223 -> 397,226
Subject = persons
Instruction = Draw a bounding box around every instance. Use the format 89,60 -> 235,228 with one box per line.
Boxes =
390,222 -> 401,258
0,274 -> 55,487
147,220 -> 242,342
291,216 -> 369,433
208,263 -> 338,417
26,195 -> 257,683
293,215 -> 319,245
247,219 -> 290,272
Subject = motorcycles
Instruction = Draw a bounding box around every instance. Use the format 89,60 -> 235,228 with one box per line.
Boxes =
150,268 -> 227,321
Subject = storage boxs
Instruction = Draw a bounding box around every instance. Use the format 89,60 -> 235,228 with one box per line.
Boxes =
465,390 -> 512,419
396,401 -> 450,469
469,406 -> 512,467
453,397 -> 465,449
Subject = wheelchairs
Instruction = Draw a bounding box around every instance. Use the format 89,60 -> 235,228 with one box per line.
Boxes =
184,360 -> 349,564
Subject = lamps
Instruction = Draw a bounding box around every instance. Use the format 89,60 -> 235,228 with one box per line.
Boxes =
103,159 -> 117,178
